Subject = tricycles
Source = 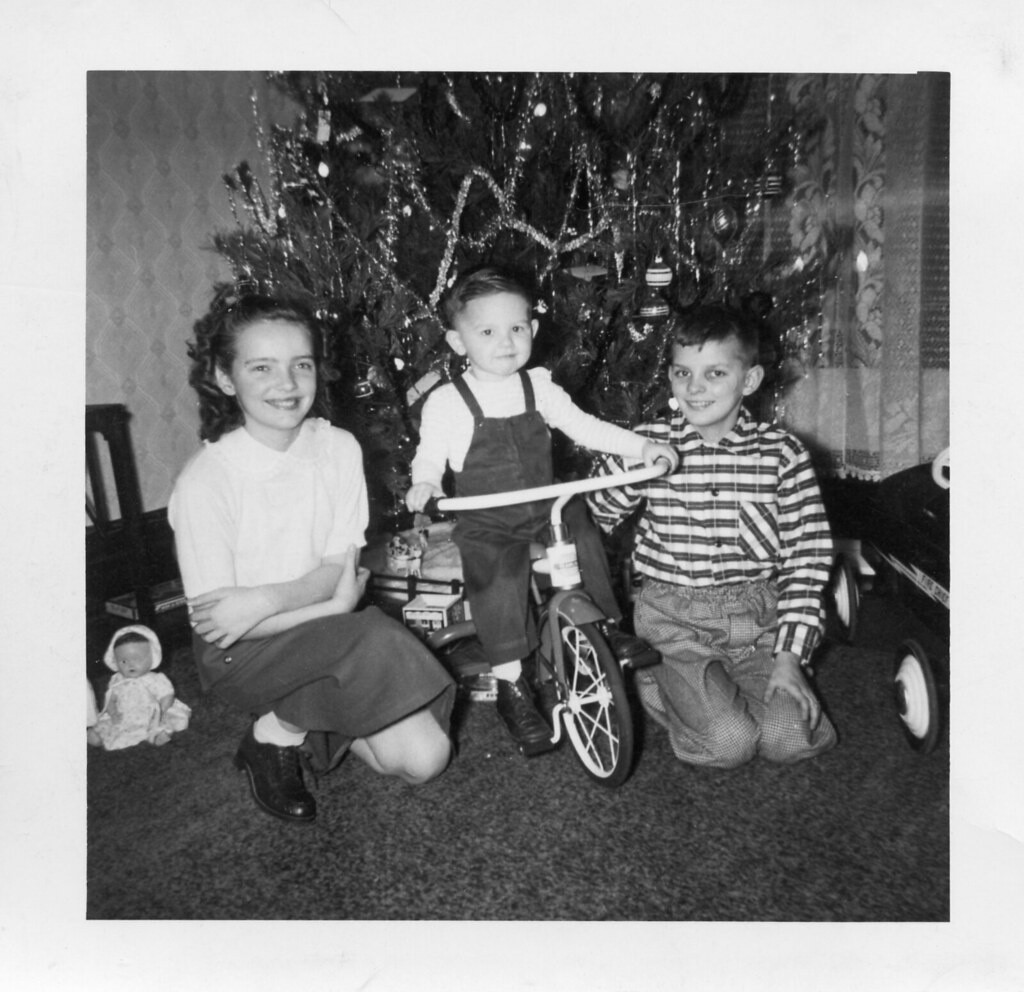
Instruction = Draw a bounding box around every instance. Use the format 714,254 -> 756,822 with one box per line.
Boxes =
357,456 -> 663,788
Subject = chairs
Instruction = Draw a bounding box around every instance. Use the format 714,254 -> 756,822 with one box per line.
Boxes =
86,404 -> 193,650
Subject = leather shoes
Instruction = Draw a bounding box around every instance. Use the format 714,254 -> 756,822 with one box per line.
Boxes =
601,620 -> 645,658
232,722 -> 321,820
495,672 -> 553,744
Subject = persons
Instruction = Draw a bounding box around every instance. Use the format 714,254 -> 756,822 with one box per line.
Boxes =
585,302 -> 836,770
407,263 -> 679,757
87,624 -> 191,750
166,295 -> 457,820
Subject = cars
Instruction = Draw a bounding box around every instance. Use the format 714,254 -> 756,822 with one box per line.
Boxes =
826,462 -> 950,755
